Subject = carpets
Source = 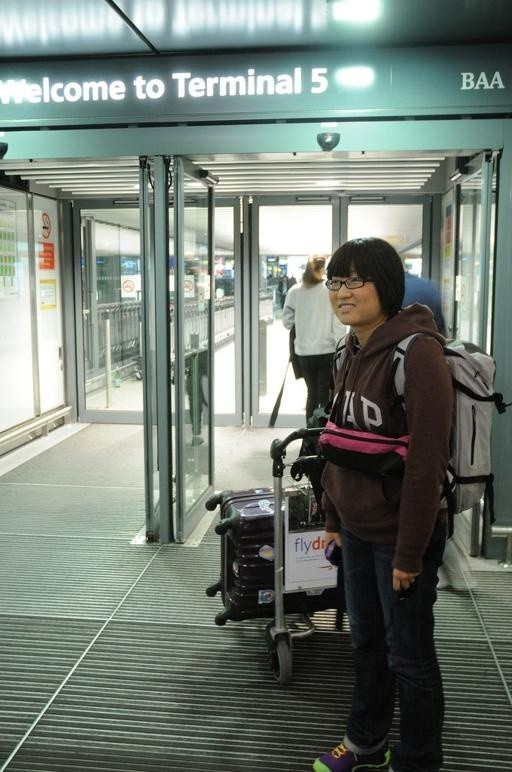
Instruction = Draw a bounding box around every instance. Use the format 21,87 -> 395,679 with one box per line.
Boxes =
0,421 -> 512,687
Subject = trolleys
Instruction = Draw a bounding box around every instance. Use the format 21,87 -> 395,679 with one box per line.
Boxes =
265,428 -> 346,684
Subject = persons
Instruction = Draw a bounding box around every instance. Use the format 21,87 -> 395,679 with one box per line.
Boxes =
279,274 -> 289,310
281,253 -> 347,425
289,274 -> 297,286
401,267 -> 449,340
312,235 -> 453,772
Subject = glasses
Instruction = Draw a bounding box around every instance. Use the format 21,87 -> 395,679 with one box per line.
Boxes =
325,276 -> 371,292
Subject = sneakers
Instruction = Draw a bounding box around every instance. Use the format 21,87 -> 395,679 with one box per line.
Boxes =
311,741 -> 392,772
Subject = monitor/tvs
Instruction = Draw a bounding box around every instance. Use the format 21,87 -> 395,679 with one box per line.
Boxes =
80,255 -> 174,276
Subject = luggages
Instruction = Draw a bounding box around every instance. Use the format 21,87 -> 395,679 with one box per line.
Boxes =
206,485 -> 346,628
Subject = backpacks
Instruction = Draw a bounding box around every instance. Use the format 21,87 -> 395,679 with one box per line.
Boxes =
331,333 -> 500,515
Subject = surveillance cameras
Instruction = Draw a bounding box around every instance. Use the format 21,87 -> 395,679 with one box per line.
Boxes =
317,132 -> 340,152
